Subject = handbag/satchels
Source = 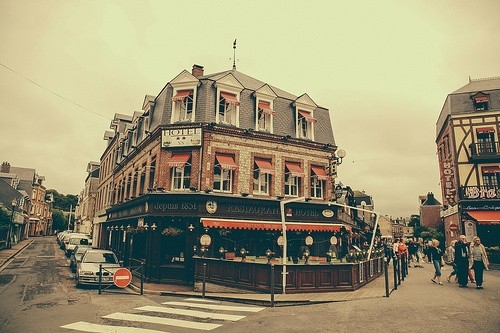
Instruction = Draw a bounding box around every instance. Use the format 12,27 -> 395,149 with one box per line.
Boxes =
440,256 -> 444,267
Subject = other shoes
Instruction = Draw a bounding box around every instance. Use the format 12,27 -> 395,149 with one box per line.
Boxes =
438,281 -> 443,285
480,285 -> 483,289
431,279 -> 437,283
458,283 -> 462,287
447,279 -> 450,283
464,283 -> 468,287
471,280 -> 476,283
477,284 -> 480,289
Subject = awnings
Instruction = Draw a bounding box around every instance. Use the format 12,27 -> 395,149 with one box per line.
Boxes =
475,97 -> 488,103
286,163 -> 329,180
298,111 -> 317,124
466,210 -> 500,224
477,127 -> 495,133
172,90 -> 193,106
259,103 -> 275,115
482,166 -> 500,173
138,111 -> 149,122
213,156 -> 238,171
254,160 -> 275,175
138,158 -> 156,174
168,154 -> 191,168
220,92 -> 240,112
200,217 -> 342,232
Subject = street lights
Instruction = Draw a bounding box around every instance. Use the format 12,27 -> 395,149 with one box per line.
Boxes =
6,200 -> 17,249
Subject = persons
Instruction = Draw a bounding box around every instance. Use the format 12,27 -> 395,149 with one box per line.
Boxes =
378,237 -> 432,271
469,236 -> 489,289
455,235 -> 469,288
431,239 -> 443,285
447,240 -> 457,284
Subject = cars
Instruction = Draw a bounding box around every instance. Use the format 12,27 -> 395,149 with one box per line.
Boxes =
56,231 -> 91,257
75,249 -> 124,288
71,245 -> 98,273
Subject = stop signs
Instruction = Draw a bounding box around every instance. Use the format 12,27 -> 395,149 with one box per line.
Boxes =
449,224 -> 458,232
113,268 -> 133,288
